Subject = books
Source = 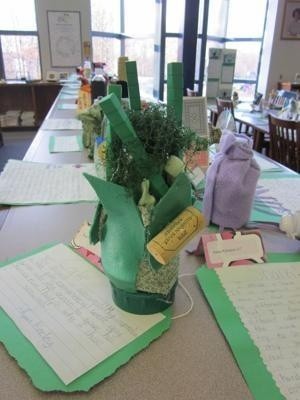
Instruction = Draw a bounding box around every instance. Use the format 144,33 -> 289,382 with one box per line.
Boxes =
0,110 -> 35,127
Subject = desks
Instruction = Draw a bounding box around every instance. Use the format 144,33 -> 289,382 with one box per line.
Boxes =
1,85 -> 300,400
0,79 -> 63,132
207,104 -> 299,153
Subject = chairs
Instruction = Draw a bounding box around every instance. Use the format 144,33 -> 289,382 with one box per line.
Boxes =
214,96 -> 234,120
268,113 -> 300,174
186,87 -> 196,97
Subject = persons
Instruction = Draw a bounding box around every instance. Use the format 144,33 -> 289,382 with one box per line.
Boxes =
286,8 -> 300,35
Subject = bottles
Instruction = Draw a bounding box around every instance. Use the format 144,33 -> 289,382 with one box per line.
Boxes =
91,63 -> 107,105
94,135 -> 107,180
84,61 -> 91,79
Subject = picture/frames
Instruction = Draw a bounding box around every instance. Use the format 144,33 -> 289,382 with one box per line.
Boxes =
47,11 -> 83,68
280,0 -> 300,40
